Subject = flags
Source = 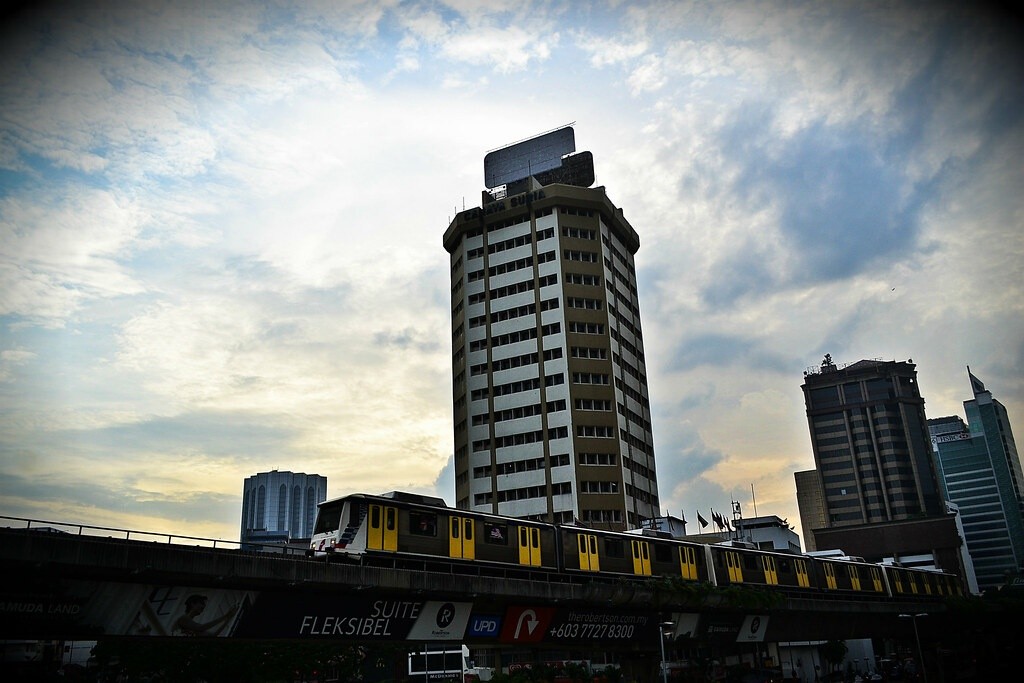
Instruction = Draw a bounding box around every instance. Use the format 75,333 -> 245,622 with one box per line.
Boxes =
667,516 -> 675,531
712,511 -> 733,533
683,515 -> 687,532
698,513 -> 708,528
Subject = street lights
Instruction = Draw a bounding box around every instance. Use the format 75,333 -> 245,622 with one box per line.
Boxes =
864,657 -> 869,671
658,621 -> 675,683
898,613 -> 929,683
853,658 -> 859,672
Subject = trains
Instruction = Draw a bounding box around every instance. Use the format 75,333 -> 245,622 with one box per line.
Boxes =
306,490 -> 970,600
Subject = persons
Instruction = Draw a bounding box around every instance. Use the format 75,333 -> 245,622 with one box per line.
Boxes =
171,594 -> 235,637
